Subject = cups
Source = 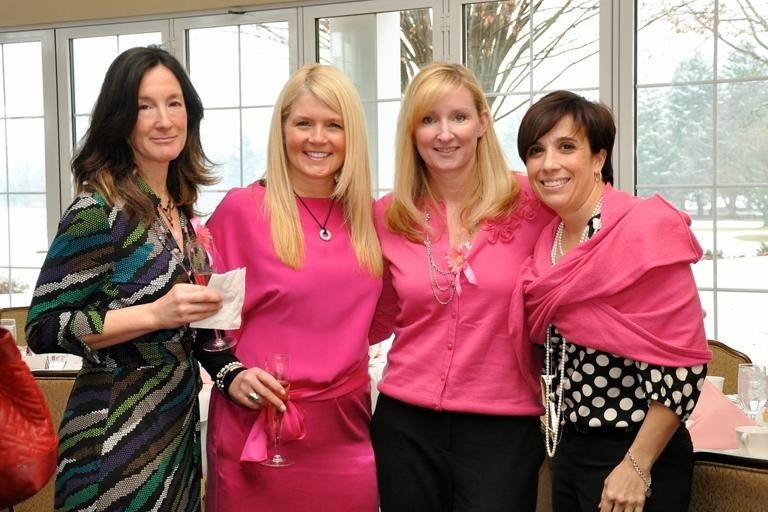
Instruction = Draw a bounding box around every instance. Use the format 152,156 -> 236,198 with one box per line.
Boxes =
0,318 -> 17,343
735,425 -> 768,460
705,376 -> 725,391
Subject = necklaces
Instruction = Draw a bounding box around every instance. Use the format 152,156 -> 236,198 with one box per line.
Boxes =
417,195 -> 474,309
158,194 -> 177,229
549,194 -> 605,266
283,180 -> 342,241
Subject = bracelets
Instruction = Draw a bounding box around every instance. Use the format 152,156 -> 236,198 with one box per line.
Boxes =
216,361 -> 244,400
624,446 -> 655,500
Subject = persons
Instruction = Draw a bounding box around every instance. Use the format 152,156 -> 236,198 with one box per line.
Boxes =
517,89 -> 713,512
365,60 -> 556,512
27,47 -> 221,510
200,64 -> 387,510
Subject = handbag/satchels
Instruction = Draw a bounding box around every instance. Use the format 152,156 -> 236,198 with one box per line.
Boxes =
0,326 -> 60,506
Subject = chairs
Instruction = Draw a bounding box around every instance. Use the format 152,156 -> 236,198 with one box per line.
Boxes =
692,449 -> 767,512
0,307 -> 28,348
704,340 -> 752,395
27,368 -> 77,433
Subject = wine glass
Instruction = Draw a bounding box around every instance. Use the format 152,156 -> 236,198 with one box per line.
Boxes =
188,237 -> 238,354
258,351 -> 292,468
737,363 -> 768,423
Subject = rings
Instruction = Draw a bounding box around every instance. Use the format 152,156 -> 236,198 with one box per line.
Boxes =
246,390 -> 261,403
